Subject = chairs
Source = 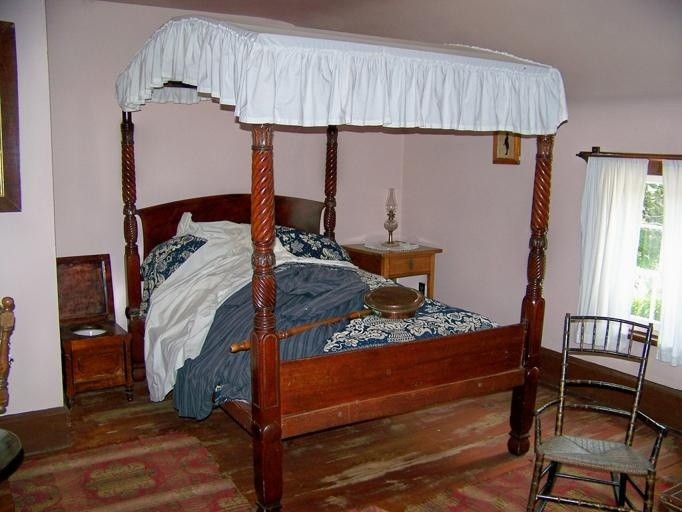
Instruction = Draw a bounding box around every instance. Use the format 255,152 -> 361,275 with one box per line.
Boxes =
527,313 -> 668,512
56,254 -> 134,409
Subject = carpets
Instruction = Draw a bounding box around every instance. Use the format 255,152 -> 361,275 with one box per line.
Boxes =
0,430 -> 257,512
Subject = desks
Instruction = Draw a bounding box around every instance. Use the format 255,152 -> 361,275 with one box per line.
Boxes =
340,241 -> 443,298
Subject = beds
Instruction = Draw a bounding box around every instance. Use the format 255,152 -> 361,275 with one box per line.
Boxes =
115,13 -> 567,512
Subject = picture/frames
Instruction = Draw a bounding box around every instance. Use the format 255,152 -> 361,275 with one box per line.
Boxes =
492,130 -> 521,165
0,21 -> 21,211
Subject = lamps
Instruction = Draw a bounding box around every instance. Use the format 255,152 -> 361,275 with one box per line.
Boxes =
382,187 -> 400,248
